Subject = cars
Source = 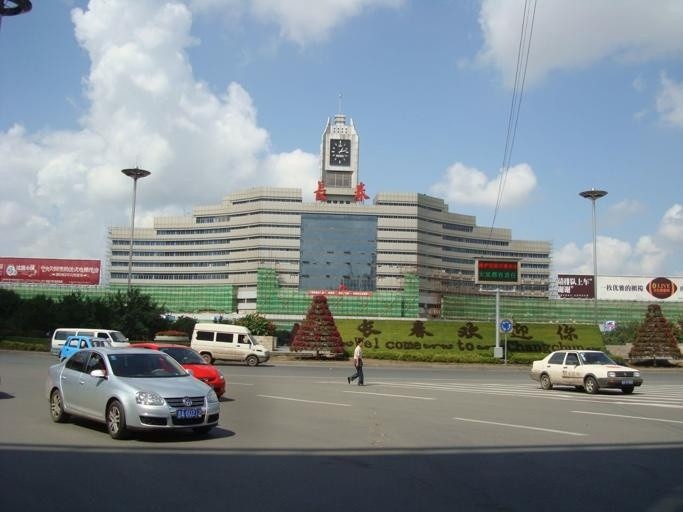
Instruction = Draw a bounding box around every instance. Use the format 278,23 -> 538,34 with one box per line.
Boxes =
59,335 -> 111,364
42,345 -> 222,441
528,349 -> 645,398
125,340 -> 227,401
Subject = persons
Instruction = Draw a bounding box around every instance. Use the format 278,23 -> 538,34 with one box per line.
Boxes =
348,338 -> 364,386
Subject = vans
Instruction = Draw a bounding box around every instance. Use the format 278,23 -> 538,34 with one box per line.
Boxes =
47,327 -> 131,358
189,321 -> 270,368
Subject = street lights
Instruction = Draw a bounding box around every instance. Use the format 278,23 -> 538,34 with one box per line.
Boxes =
576,185 -> 608,329
119,164 -> 152,290
0,0 -> 33,25
